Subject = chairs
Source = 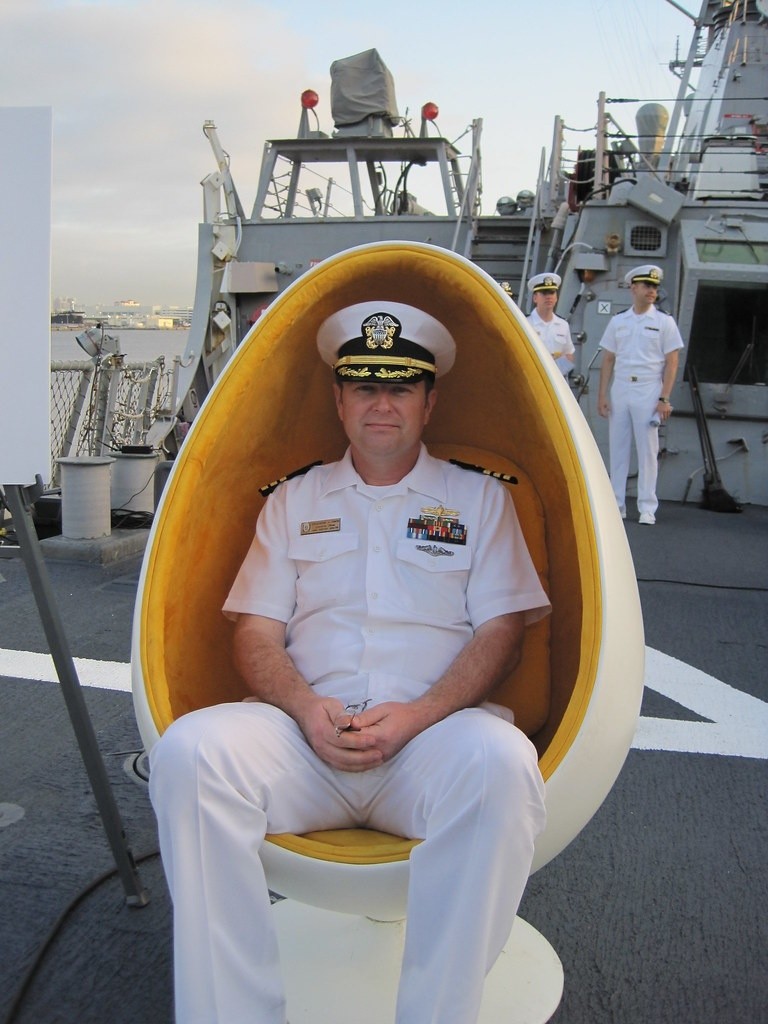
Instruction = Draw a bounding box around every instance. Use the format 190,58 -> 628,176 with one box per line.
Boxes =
132,238 -> 644,1024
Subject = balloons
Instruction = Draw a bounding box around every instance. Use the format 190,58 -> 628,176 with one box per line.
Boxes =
302,90 -> 318,109
423,102 -> 438,120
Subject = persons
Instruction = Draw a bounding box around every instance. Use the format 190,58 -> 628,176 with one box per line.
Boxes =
526,272 -> 575,385
148,301 -> 546,1024
597,265 -> 685,524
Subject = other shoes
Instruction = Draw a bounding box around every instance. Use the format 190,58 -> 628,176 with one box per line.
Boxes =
639,513 -> 655,525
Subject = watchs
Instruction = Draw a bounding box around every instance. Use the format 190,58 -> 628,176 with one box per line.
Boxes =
659,397 -> 670,403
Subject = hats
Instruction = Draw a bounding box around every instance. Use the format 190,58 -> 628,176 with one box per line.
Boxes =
497,281 -> 513,295
624,265 -> 664,284
526,273 -> 561,293
317,301 -> 456,384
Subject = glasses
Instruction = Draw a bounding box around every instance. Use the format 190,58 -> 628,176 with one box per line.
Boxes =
333,697 -> 373,737
